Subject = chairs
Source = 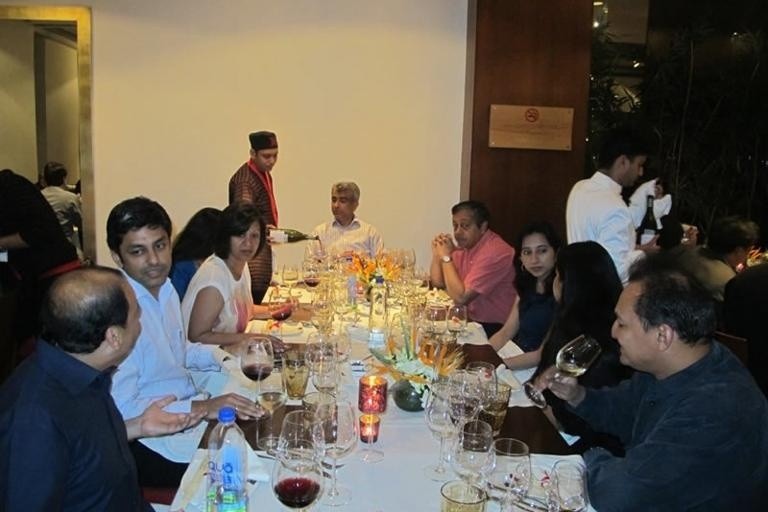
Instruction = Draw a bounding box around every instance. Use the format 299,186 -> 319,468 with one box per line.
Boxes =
708,327 -> 751,365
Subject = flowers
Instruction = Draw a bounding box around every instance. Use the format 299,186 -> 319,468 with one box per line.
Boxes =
363,319 -> 468,397
342,240 -> 403,301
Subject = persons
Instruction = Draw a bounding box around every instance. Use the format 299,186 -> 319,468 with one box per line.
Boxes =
0,267 -> 207,512
564,126 -> 668,287
102,197 -> 294,491
429,198 -> 517,340
489,217 -> 582,369
304,181 -> 386,276
543,257 -> 768,509
227,132 -> 279,304
662,217 -> 767,393
1,161 -> 81,375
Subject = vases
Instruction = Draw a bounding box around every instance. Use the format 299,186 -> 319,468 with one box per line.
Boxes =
392,377 -> 432,413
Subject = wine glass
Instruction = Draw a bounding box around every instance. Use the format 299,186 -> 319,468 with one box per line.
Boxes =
235,241 -> 589,511
523,332 -> 602,407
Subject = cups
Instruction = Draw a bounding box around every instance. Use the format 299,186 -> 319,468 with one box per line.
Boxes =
480,377 -> 512,432
358,415 -> 380,443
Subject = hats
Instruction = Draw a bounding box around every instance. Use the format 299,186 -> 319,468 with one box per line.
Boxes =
249,132 -> 277,150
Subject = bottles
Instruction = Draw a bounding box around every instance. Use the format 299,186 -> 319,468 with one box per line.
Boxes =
638,195 -> 658,248
266,226 -> 323,243
203,407 -> 249,512
